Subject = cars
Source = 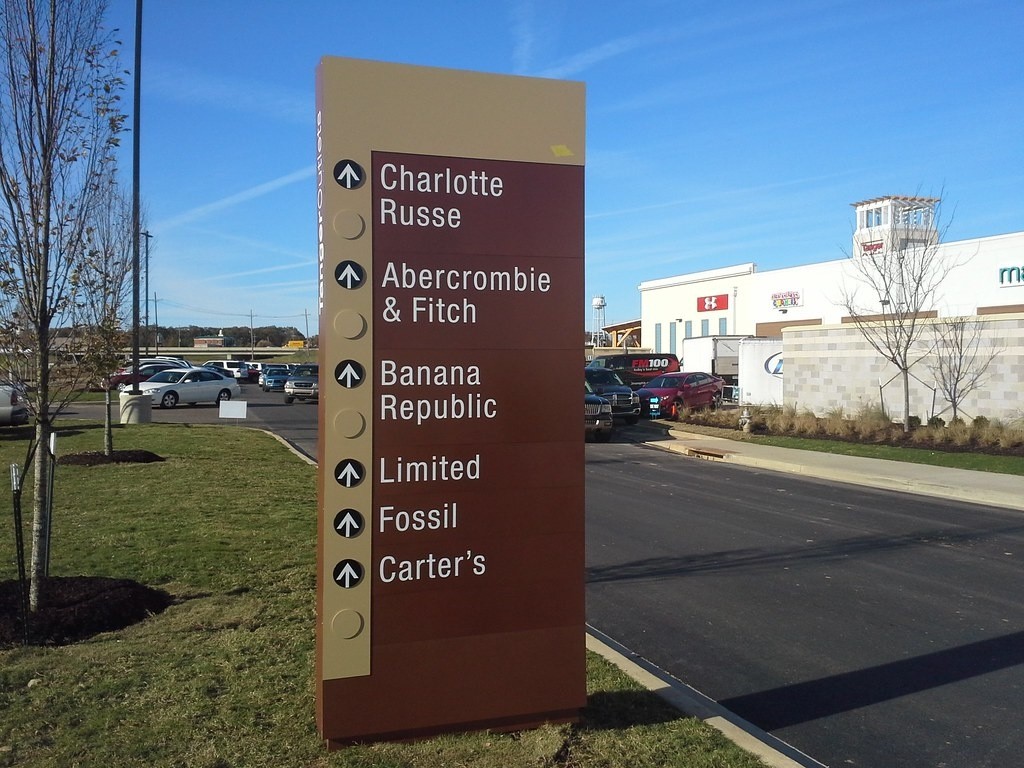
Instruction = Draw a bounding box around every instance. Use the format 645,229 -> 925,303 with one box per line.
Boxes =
635,372 -> 725,419
263,369 -> 292,392
193,365 -> 234,378
584,380 -> 613,438
237,363 -> 260,382
101,356 -> 193,392
258,369 -> 282,387
118,368 -> 240,409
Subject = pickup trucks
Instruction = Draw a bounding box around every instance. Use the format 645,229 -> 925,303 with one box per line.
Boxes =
0,384 -> 30,425
50,355 -> 85,373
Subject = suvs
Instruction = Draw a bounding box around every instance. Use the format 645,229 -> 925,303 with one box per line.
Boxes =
267,364 -> 295,374
585,366 -> 641,422
202,360 -> 248,379
245,362 -> 267,373
283,364 -> 319,404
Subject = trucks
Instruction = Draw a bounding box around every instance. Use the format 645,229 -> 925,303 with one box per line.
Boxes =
679,335 -> 755,400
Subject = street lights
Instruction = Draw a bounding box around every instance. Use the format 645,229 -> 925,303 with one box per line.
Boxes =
141,232 -> 154,356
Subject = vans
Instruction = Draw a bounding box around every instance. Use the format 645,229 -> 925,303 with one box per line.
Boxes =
589,352 -> 682,383
